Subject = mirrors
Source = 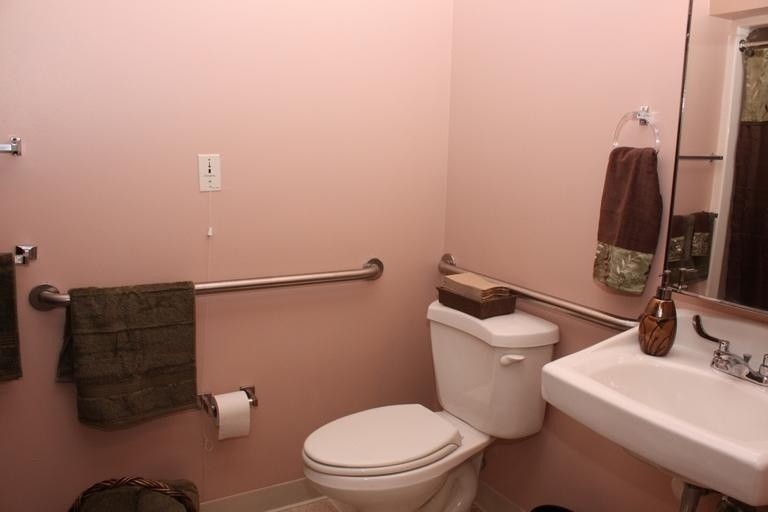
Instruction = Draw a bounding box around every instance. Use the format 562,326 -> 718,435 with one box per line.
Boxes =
661,0 -> 768,331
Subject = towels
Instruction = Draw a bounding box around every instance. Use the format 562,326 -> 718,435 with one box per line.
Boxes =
590,147 -> 662,299
665,214 -> 694,287
692,209 -> 718,285
724,27 -> 768,308
53,279 -> 203,432
1,251 -> 26,386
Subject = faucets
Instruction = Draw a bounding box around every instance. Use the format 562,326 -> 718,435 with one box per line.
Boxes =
716,353 -> 768,386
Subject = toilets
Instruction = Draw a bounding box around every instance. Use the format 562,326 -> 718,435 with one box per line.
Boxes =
301,297 -> 560,512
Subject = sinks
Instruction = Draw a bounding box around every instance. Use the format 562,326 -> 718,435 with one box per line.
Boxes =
540,307 -> 768,508
